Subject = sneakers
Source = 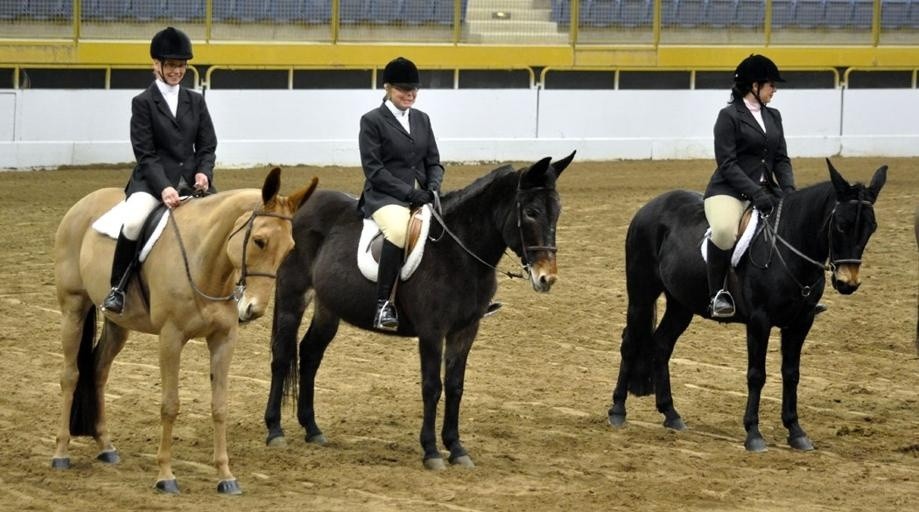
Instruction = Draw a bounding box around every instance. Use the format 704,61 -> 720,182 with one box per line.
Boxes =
105,224 -> 137,313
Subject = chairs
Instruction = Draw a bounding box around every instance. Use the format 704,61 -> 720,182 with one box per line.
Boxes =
551,0 -> 919,31
0,0 -> 468,24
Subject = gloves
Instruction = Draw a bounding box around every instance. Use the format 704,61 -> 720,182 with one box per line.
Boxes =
753,189 -> 778,212
427,182 -> 440,195
406,188 -> 431,206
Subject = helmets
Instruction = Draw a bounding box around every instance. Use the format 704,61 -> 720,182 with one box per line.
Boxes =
383,57 -> 423,89
734,55 -> 787,82
151,27 -> 192,60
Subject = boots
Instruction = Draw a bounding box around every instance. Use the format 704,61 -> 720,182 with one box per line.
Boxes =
373,238 -> 405,329
707,238 -> 737,313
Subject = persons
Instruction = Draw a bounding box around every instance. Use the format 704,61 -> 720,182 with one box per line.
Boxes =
358,57 -> 503,336
105,26 -> 217,315
702,54 -> 826,321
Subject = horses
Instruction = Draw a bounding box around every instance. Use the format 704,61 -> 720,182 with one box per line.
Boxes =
52,162 -> 321,498
261,147 -> 578,479
605,155 -> 889,455
